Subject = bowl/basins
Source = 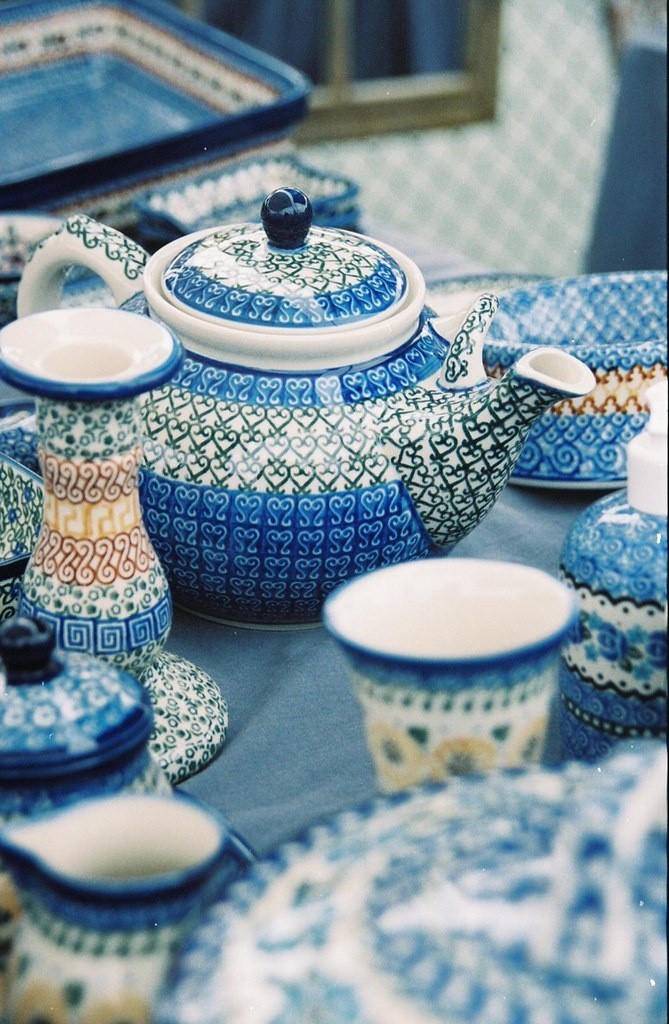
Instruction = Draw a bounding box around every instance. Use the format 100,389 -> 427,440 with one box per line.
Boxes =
482,270 -> 669,491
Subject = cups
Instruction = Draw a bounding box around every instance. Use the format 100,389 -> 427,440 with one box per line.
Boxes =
322,557 -> 580,795
0,618 -> 177,974
0,788 -> 259,1024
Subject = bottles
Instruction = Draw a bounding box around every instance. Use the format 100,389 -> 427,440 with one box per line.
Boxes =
559,379 -> 669,766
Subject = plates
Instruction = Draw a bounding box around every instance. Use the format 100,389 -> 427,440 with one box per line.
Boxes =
0,452 -> 44,567
427,272 -> 560,317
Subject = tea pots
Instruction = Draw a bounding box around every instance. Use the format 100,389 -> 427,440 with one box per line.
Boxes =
16,188 -> 595,632
